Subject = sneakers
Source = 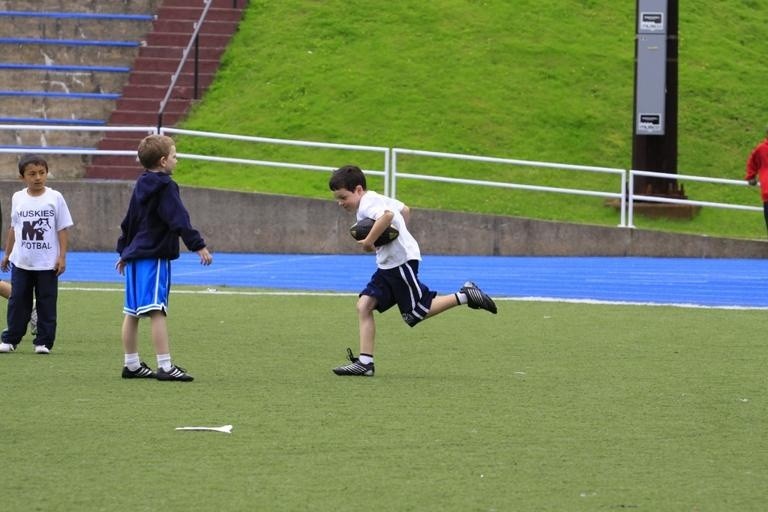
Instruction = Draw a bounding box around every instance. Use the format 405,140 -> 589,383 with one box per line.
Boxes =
460,281 -> 497,314
35,344 -> 49,354
0,342 -> 14,352
122,362 -> 193,381
333,348 -> 374,376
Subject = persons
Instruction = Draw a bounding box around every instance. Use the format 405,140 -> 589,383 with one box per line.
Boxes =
745,129 -> 768,230
115,134 -> 212,381
330,165 -> 497,376
0,202 -> 40,335
0,153 -> 74,353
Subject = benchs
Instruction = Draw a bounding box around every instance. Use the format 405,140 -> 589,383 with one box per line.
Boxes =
1,1 -> 164,170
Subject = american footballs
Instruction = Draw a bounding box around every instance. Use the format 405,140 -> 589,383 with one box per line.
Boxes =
349,217 -> 400,247
